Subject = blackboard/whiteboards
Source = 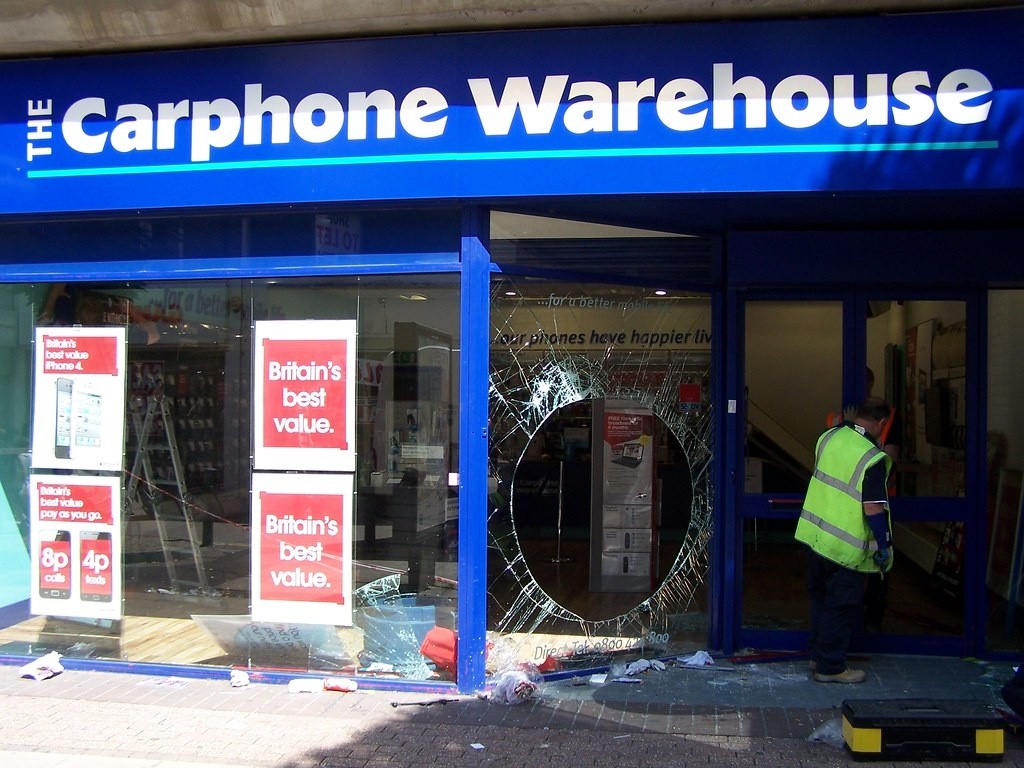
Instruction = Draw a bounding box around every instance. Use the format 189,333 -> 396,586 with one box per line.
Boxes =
987,467 -> 1023,602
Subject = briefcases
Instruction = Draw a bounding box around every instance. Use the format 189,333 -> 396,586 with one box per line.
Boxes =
843,698 -> 1005,762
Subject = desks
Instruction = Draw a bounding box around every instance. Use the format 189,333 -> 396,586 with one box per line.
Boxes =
355,486 -> 443,591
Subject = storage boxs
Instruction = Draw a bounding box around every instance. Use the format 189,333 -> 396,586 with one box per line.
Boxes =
840,698 -> 1006,763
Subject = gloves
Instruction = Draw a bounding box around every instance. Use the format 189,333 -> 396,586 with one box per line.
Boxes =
863,509 -> 893,572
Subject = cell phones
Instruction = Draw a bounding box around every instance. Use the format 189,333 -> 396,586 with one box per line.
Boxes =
56,378 -> 108,459
80,531 -> 113,602
40,529 -> 71,600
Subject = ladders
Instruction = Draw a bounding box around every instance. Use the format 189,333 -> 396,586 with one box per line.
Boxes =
124,392 -> 211,597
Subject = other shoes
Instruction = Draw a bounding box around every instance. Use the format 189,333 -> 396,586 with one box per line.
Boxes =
814,666 -> 866,683
808,660 -> 817,669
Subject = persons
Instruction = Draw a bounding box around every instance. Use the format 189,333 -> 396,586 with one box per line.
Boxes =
825,367 -> 903,463
794,394 -> 894,683
